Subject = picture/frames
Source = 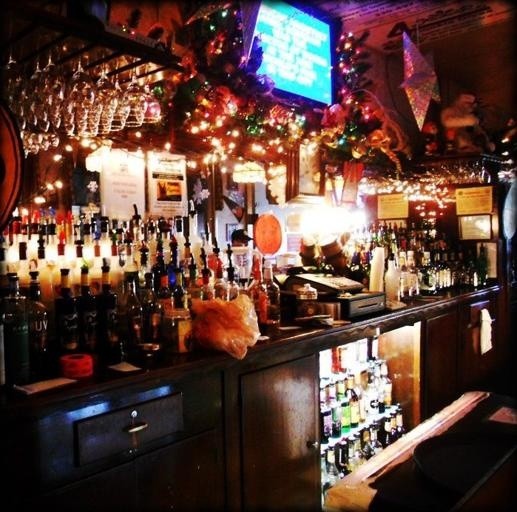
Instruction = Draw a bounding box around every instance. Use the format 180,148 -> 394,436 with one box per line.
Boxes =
458,213 -> 493,241
377,192 -> 410,220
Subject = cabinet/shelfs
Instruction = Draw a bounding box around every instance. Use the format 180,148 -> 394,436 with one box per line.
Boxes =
2,370 -> 227,511
241,298 -> 497,510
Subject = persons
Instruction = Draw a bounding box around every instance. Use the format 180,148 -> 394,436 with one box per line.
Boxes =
231,229 -> 253,246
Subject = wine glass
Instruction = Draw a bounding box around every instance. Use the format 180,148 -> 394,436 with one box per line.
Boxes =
417,157 -> 491,187
0,48 -> 162,156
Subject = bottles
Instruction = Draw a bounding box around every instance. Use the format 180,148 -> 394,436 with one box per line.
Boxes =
320,357 -> 405,484
357,219 -> 489,305
1,216 -> 280,372
297,283 -> 321,320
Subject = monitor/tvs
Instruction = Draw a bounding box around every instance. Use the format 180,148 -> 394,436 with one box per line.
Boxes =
239,0 -> 335,112
